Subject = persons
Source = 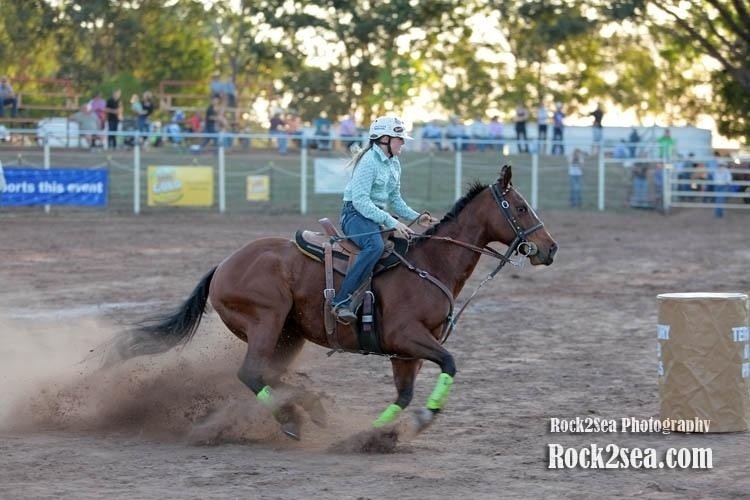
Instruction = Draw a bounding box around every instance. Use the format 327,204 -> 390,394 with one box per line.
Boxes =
614,127 -> 733,217
267,108 -> 356,154
590,100 -> 605,155
568,147 -> 584,207
68,87 -> 251,154
536,100 -> 547,154
0,75 -> 17,117
551,101 -> 565,155
331,114 -> 438,325
425,114 -> 504,151
514,99 -> 529,153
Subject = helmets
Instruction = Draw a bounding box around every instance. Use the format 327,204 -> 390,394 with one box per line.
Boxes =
368,115 -> 415,141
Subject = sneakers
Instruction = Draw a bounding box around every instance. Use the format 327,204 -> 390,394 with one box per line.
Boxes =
330,304 -> 358,325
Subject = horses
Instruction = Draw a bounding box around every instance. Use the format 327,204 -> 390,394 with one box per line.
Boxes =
87,164 -> 558,445
